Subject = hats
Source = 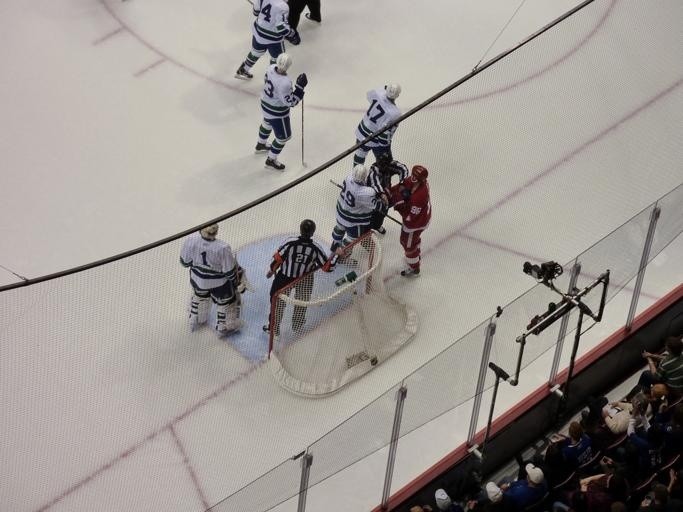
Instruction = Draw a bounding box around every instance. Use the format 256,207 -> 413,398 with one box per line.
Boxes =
485,481 -> 503,504
433,487 -> 453,511
525,463 -> 545,484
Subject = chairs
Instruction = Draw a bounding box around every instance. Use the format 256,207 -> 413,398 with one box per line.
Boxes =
409,438 -> 568,511
568,327 -> 682,511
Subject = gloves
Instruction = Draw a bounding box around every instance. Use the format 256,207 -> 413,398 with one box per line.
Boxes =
293,73 -> 309,91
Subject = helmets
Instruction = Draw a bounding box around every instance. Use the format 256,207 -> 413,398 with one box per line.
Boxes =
351,164 -> 367,183
199,223 -> 220,239
383,81 -> 403,102
275,51 -> 293,73
411,165 -> 429,183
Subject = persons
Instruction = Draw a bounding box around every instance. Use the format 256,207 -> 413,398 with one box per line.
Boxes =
237,2 -> 296,78
263,220 -> 344,335
391,165 -> 431,277
283,1 -> 321,44
179,223 -> 239,333
256,54 -> 308,171
367,152 -> 409,234
353,83 -> 401,169
332,164 -> 382,257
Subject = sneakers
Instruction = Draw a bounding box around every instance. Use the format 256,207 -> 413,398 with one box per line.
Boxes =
262,323 -> 280,337
303,12 -> 321,23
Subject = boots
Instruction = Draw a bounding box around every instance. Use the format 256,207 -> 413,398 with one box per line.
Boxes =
187,315 -> 245,336
235,61 -> 253,80
264,156 -> 286,170
401,265 -> 421,276
255,141 -> 272,151
291,318 -> 306,331
337,256 -> 358,265
374,225 -> 387,234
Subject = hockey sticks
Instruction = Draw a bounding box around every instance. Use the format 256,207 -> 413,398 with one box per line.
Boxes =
302,97 -> 310,167
